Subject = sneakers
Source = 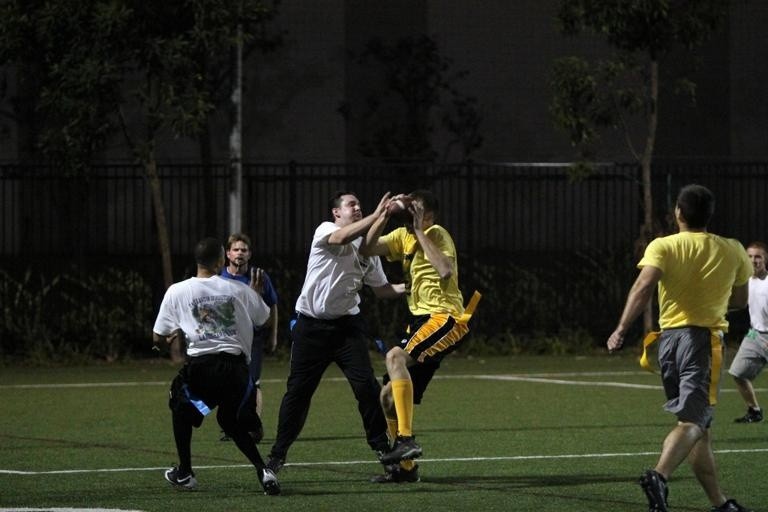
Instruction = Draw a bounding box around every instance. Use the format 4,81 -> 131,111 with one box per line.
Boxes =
639,470 -> 670,512
735,407 -> 763,423
713,499 -> 749,512
379,436 -> 423,463
258,468 -> 281,495
267,454 -> 284,473
373,466 -> 421,485
164,463 -> 199,491
376,449 -> 403,474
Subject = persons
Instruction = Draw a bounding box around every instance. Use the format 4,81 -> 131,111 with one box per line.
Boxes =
727,244 -> 768,423
152,239 -> 279,498
610,182 -> 754,511
219,233 -> 278,443
360,192 -> 481,482
265,193 -> 392,473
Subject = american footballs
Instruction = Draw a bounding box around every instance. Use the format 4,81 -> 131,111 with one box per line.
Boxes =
386,195 -> 414,215
